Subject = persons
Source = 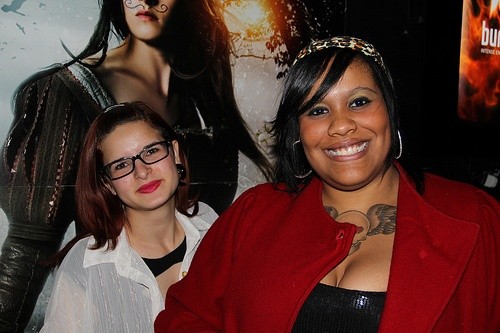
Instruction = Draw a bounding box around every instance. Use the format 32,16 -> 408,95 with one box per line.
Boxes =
38,100 -> 220,333
152,34 -> 500,333
0,0 -> 273,333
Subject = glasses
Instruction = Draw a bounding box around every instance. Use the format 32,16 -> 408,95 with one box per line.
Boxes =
102,139 -> 171,180
